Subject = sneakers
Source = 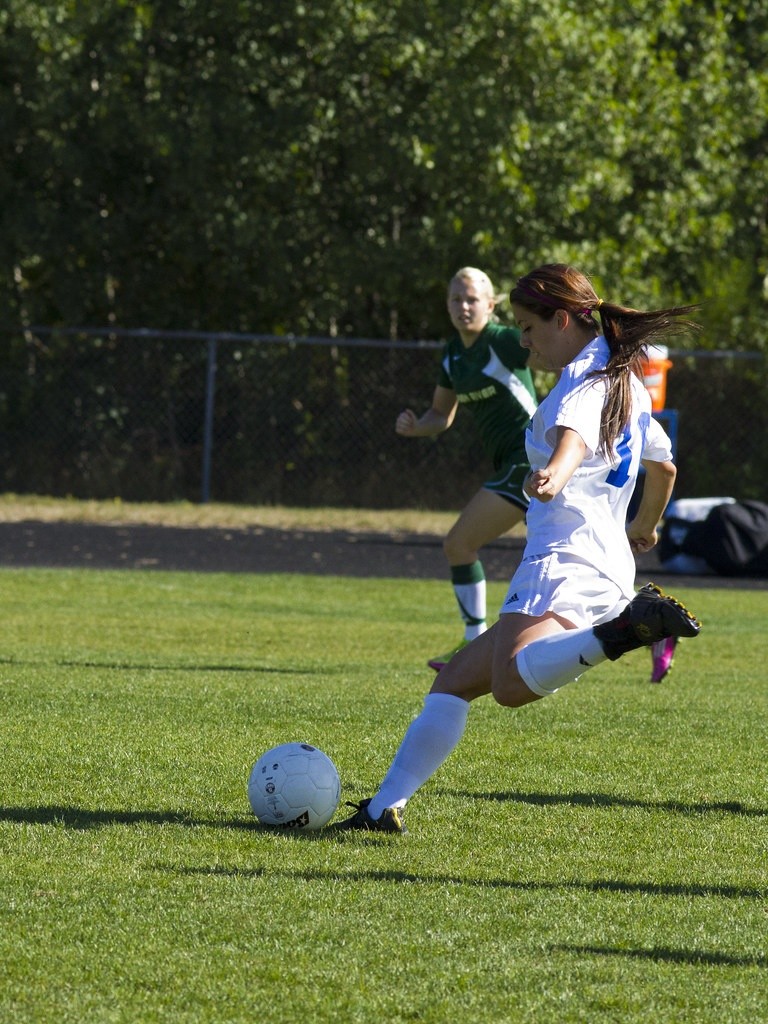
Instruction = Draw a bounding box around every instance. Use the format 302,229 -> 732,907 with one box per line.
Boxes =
649,635 -> 679,684
620,582 -> 703,648
322,798 -> 411,840
428,639 -> 474,673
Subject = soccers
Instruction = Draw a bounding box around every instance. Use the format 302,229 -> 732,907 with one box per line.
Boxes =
248,741 -> 343,836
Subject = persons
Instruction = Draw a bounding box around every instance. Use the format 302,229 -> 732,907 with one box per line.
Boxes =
396,265 -> 681,685
327,264 -> 700,835
